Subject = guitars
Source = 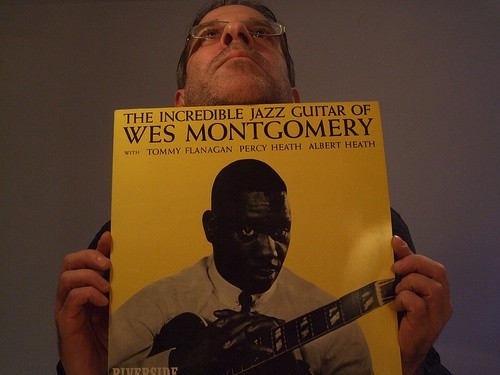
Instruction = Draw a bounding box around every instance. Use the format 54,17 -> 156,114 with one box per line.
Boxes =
147,278 -> 395,374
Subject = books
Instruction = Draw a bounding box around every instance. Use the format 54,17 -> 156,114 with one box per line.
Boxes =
109,99 -> 399,375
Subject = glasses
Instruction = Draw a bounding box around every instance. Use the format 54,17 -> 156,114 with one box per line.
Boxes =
181,20 -> 292,75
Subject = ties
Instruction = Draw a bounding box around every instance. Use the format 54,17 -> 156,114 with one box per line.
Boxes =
238,292 -> 257,312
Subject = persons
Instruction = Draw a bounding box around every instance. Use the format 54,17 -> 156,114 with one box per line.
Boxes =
55,2 -> 454,375
111,159 -> 373,375
